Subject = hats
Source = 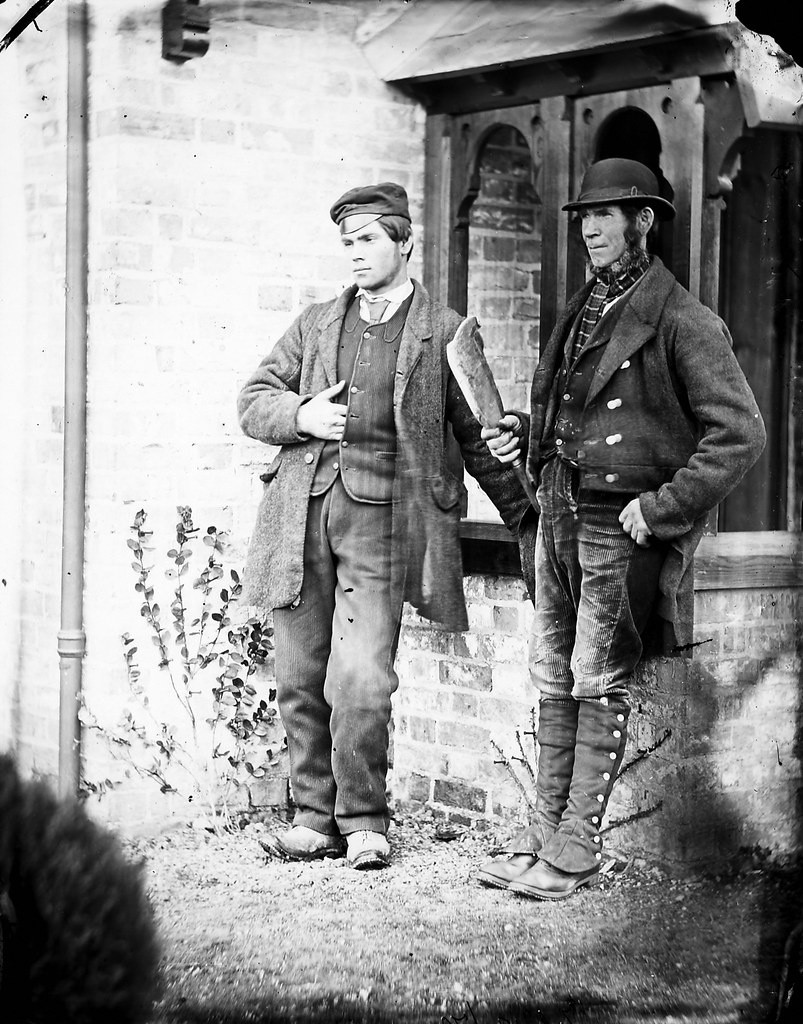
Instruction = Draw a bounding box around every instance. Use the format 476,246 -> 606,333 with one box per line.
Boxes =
561,158 -> 676,222
330,182 -> 411,233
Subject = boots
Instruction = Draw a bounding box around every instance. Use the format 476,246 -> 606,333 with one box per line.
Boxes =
507,702 -> 631,901
476,699 -> 580,889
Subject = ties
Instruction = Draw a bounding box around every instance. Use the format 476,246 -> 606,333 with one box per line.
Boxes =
365,299 -> 390,324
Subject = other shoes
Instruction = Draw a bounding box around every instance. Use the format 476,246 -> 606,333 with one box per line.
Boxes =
346,830 -> 390,870
258,826 -> 346,862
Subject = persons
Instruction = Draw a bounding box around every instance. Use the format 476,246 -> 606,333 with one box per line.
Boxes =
237,182 -> 531,870
481,160 -> 767,900
